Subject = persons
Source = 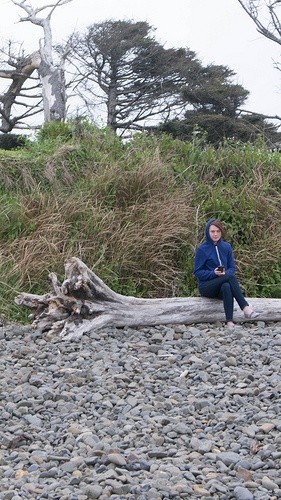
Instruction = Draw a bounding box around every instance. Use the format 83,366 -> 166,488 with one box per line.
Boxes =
194,218 -> 261,331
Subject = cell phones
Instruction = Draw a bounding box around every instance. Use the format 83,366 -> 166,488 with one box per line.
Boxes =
217,265 -> 225,273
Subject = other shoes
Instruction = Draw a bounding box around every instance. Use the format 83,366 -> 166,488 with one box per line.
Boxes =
242,311 -> 260,319
